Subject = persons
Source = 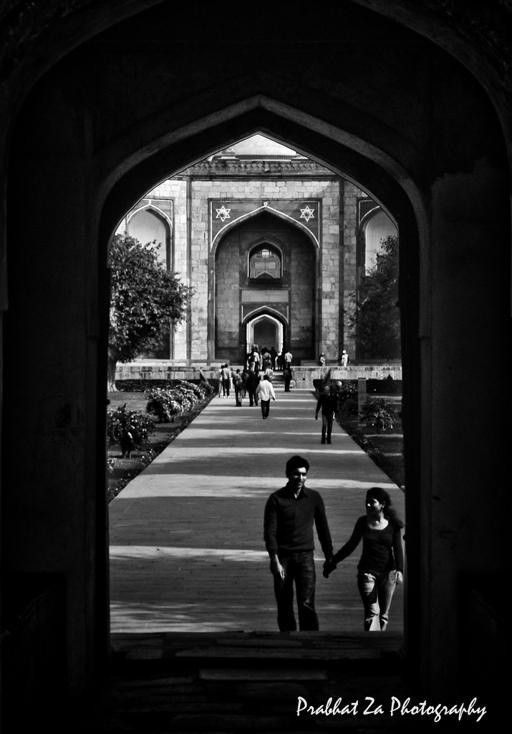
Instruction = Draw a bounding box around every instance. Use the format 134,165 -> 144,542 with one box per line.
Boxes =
340,349 -> 349,366
262,454 -> 335,632
319,352 -> 327,366
217,344 -> 294,407
314,384 -> 337,444
255,373 -> 276,420
323,487 -> 405,633
